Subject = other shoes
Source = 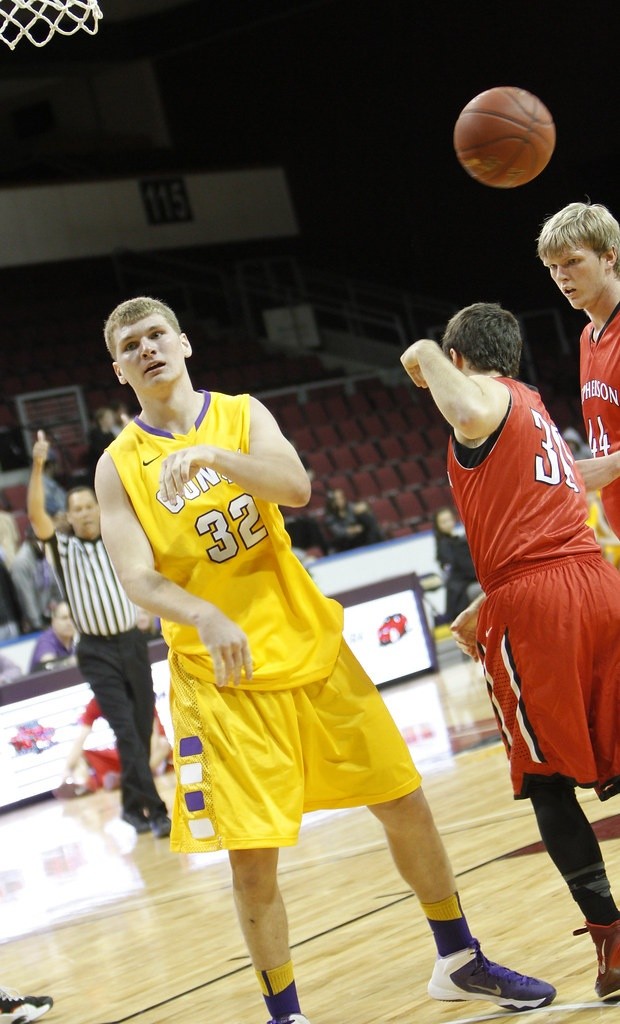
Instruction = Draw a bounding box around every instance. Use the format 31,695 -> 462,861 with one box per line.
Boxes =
122,812 -> 152,834
149,814 -> 171,837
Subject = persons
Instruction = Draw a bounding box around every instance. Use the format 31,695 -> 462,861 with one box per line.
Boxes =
537,204 -> 620,542
53,694 -> 172,800
83,407 -> 132,481
284,514 -> 326,562
324,488 -> 386,552
0,493 -> 80,686
432,508 -> 484,619
401,304 -> 620,1005
29,430 -> 172,839
562,427 -> 592,461
93,298 -> 557,1024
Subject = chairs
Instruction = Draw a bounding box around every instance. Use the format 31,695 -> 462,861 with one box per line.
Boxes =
0,297 -> 585,559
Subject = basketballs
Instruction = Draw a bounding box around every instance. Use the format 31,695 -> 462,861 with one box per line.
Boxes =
453,85 -> 557,190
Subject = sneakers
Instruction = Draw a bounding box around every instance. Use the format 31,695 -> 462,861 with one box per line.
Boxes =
427,938 -> 556,1012
266,1013 -> 310,1024
0,987 -> 53,1024
573,913 -> 620,1002
